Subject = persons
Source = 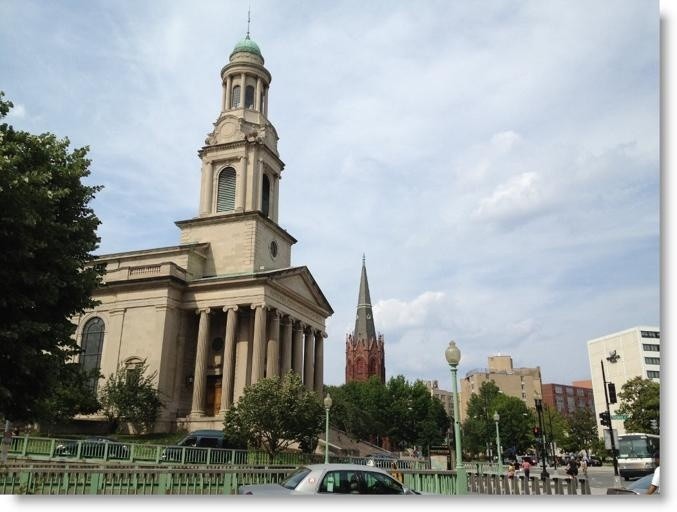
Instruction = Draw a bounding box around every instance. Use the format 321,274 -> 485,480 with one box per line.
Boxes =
514,459 -> 522,476
10,427 -> 20,451
522,459 -> 530,481
509,464 -> 515,476
565,456 -> 578,479
646,452 -> 660,495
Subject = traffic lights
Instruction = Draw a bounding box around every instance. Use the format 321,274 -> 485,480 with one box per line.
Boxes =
599,411 -> 609,426
607,383 -> 616,403
532,427 -> 539,435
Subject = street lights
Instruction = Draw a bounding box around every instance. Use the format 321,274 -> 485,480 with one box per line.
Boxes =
322,392 -> 332,465
443,341 -> 469,495
492,410 -> 504,468
532,393 -> 549,479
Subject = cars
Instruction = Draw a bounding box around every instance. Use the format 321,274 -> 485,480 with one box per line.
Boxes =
52,436 -> 127,460
360,454 -> 394,466
588,455 -> 601,466
237,463 -> 443,495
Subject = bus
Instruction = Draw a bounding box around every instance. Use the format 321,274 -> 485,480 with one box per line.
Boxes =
614,433 -> 658,480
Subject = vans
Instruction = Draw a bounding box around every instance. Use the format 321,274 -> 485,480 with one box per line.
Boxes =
161,429 -> 248,465
